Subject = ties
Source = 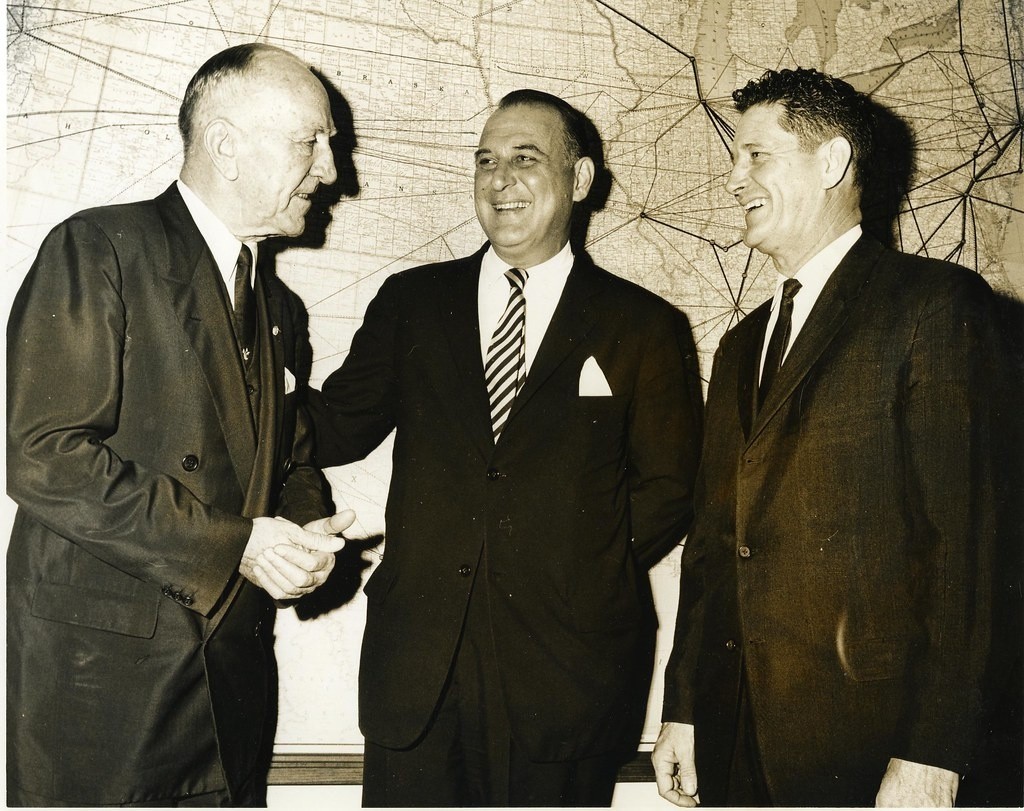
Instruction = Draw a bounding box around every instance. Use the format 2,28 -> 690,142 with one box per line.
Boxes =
483,268 -> 527,442
755,278 -> 802,392
233,244 -> 259,374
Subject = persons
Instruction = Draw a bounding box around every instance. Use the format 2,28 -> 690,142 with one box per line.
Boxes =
7,40 -> 356,807
647,66 -> 1023,807
306,88 -> 703,807
